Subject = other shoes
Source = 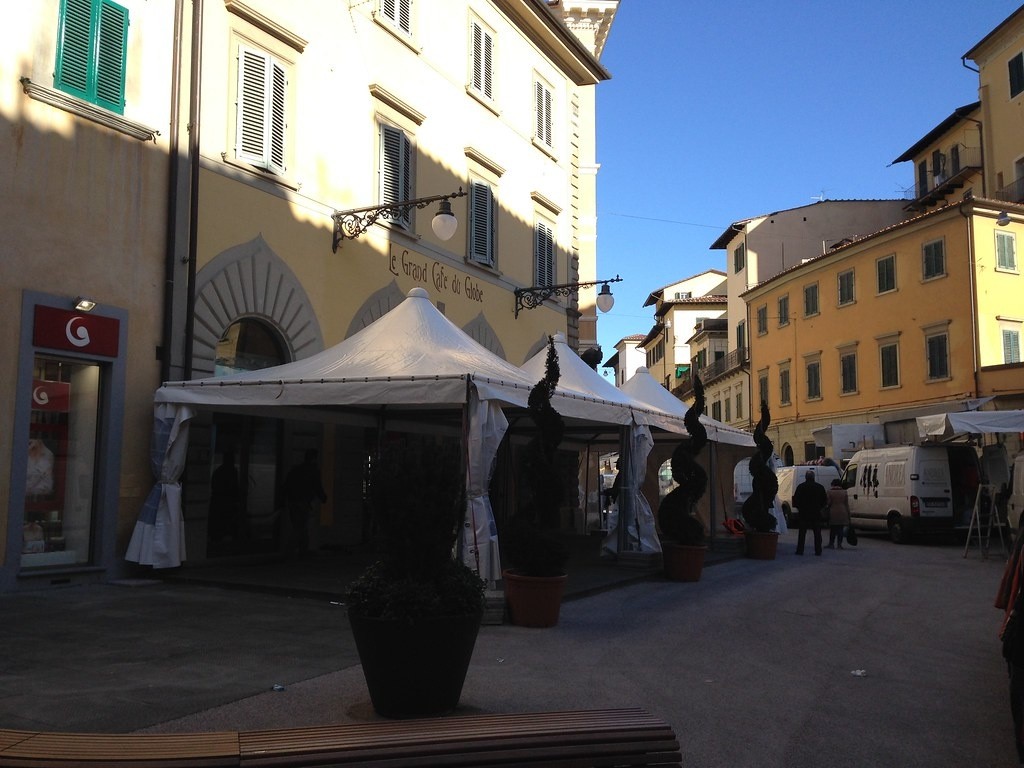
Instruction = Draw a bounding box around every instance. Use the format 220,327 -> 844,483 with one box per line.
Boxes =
815,551 -> 821,555
824,544 -> 833,549
795,549 -> 804,555
838,544 -> 843,549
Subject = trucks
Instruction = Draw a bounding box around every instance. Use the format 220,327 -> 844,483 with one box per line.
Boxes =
840,440 -> 1010,543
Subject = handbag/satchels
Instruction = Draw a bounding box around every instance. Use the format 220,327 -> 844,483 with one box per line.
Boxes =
845,526 -> 857,545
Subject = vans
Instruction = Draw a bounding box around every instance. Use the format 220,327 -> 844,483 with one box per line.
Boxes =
1006,454 -> 1024,535
775,466 -> 842,529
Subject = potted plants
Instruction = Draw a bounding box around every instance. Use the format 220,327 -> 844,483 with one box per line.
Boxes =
742,400 -> 781,559
658,374 -> 708,582
347,434 -> 488,720
502,335 -> 569,628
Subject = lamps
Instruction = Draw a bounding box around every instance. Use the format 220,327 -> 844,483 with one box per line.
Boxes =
331,186 -> 468,256
514,275 -> 623,320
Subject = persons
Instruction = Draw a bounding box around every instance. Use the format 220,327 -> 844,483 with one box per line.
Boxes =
793,470 -> 828,556
124,286 -> 758,584
823,478 -> 851,548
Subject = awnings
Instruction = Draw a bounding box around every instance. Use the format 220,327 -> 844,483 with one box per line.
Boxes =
708,224 -> 745,250
642,288 -> 662,308
812,423 -> 886,461
654,302 -> 674,316
914,408 -> 1024,439
635,324 -> 664,354
602,351 -> 619,367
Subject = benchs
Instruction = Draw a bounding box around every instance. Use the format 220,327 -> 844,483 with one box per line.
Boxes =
0,705 -> 684,768
